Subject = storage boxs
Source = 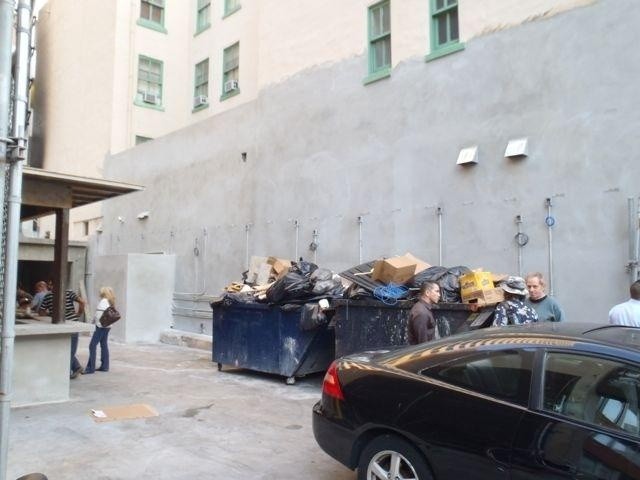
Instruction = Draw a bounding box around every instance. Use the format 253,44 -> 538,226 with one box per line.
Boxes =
265,257 -> 292,280
371,256 -> 508,303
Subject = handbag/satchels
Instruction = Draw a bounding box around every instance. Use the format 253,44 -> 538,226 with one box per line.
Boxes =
98,306 -> 121,328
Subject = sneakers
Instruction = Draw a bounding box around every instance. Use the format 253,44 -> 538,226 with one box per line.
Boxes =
71,367 -> 107,378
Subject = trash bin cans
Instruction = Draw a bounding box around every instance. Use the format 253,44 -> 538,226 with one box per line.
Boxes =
211,296 -> 336,385
335,297 -> 501,360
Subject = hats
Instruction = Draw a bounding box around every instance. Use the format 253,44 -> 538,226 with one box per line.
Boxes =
500,278 -> 529,298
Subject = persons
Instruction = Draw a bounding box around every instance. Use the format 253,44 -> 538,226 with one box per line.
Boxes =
523,271 -> 567,321
490,276 -> 539,327
406,279 -> 441,345
38,278 -> 86,379
607,279 -> 640,328
81,286 -> 116,374
19,280 -> 49,313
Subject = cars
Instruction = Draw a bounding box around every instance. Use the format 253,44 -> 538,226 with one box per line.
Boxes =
312,321 -> 640,479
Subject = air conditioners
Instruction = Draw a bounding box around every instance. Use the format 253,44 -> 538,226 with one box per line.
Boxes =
143,92 -> 156,103
225,80 -> 237,93
194,95 -> 207,107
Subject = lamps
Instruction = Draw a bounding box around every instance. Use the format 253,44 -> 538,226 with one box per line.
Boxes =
136,212 -> 148,219
456,146 -> 478,166
504,138 -> 528,159
